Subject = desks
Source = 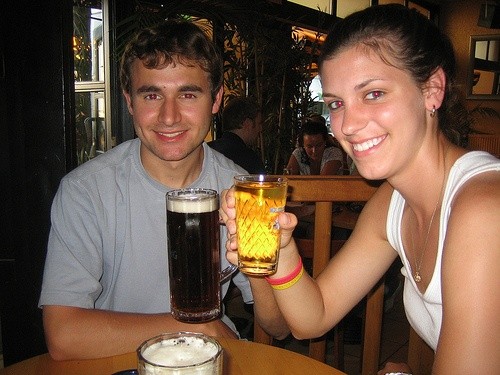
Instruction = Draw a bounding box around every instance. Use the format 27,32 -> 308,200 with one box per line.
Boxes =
0,339 -> 345,375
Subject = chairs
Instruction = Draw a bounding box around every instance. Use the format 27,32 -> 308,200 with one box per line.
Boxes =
252,177 -> 421,375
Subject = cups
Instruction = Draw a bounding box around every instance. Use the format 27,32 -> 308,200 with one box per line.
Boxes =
234,174 -> 287,276
166,188 -> 238,323
137,331 -> 224,374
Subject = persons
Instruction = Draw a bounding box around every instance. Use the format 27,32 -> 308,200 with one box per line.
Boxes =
205,97 -> 315,338
38,20 -> 291,361
283,122 -> 344,175
309,112 -> 343,148
219,3 -> 500,375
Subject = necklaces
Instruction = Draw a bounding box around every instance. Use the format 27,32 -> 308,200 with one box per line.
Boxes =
413,141 -> 454,282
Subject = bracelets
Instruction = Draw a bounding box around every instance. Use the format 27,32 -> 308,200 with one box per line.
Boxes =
264,255 -> 304,289
